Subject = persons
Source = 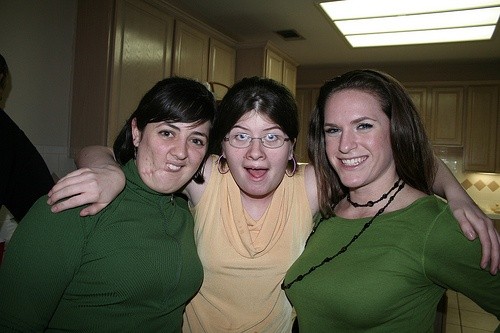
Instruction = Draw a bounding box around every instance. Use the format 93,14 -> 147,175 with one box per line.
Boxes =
0,77 -> 216,332
282,70 -> 500,333
45,75 -> 500,333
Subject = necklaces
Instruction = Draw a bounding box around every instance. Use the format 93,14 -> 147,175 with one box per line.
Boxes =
281,178 -> 405,307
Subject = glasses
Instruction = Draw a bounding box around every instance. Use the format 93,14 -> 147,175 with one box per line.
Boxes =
223,133 -> 290,149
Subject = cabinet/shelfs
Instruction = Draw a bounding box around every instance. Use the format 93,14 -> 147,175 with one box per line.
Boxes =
68,0 -> 500,173
